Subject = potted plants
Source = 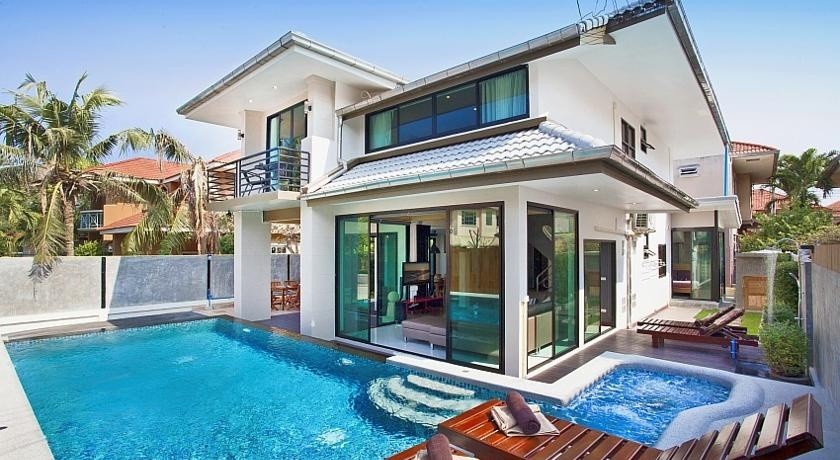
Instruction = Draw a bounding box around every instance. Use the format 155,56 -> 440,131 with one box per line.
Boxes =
762,322 -> 809,386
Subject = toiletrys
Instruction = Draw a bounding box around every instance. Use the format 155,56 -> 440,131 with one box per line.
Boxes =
730,337 -> 738,361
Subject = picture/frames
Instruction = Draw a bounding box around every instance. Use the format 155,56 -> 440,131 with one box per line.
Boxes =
402,262 -> 431,285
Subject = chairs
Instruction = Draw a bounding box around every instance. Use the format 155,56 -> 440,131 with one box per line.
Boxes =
388,440 -> 480,460
272,281 -> 299,310
637,304 -> 759,357
440,393 -> 824,460
241,170 -> 269,196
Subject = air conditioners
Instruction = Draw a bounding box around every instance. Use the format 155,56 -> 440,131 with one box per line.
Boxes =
633,213 -> 656,231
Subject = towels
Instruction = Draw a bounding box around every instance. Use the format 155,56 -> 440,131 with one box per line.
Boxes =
425,433 -> 453,460
506,390 -> 541,435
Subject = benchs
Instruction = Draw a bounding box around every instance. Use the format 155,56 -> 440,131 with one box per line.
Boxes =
672,280 -> 693,293
403,315 -> 500,362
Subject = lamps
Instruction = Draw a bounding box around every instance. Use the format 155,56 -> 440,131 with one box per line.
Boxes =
237,130 -> 244,140
431,242 -> 440,254
304,100 -> 311,112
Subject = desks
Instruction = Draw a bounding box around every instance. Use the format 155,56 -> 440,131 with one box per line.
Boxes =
273,284 -> 297,299
